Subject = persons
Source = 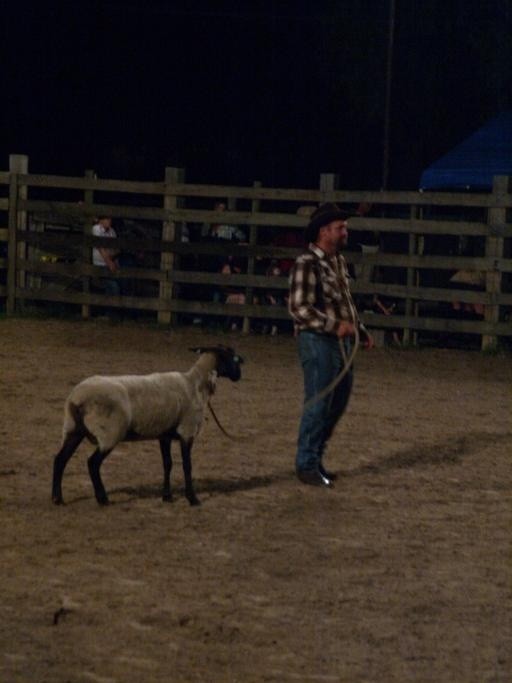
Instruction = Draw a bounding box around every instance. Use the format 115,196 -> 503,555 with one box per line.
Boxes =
86,206 -> 487,344
289,199 -> 375,492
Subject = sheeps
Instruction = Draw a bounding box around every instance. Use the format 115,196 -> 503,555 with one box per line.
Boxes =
51,342 -> 246,507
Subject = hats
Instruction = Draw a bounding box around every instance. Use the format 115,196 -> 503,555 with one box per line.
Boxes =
304,202 -> 350,242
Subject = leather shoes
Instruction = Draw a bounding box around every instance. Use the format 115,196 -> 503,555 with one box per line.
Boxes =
297,467 -> 335,487
